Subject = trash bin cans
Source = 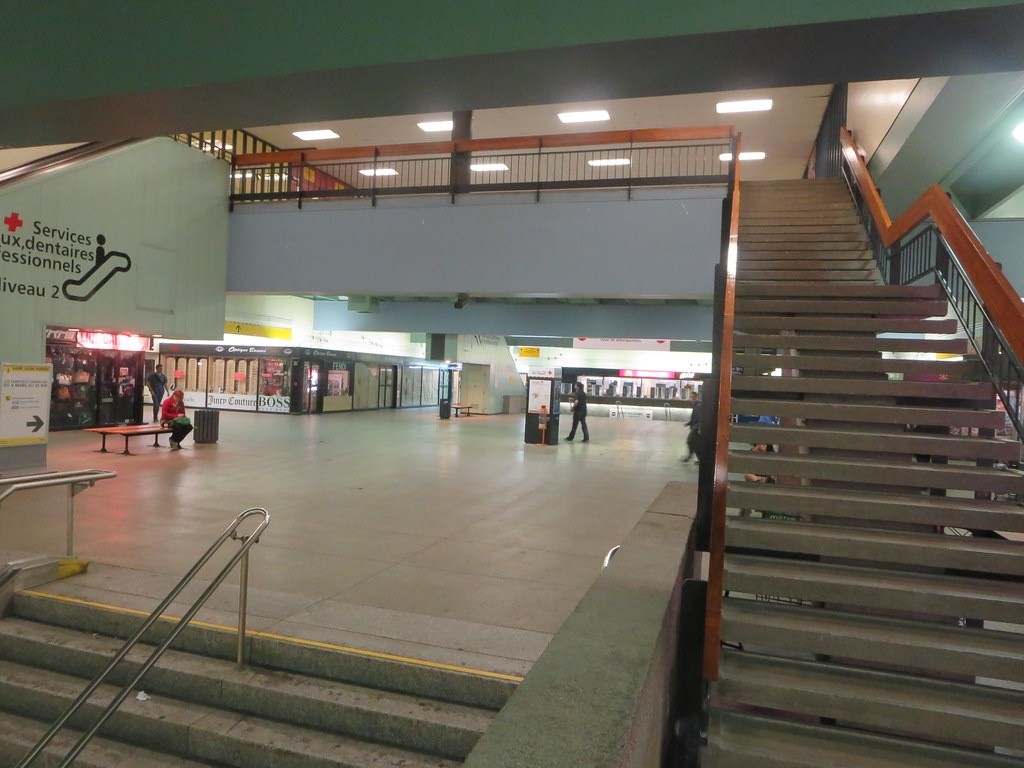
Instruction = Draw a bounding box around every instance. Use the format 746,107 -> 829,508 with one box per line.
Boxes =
194,410 -> 219,444
440,399 -> 450,419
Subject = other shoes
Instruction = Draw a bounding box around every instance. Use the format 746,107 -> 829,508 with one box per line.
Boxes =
565,437 -> 573,441
168,437 -> 179,449
177,443 -> 181,449
153,419 -> 159,423
582,439 -> 589,443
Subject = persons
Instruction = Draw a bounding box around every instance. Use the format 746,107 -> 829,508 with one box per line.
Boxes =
679,393 -> 700,464
744,443 -> 776,484
162,390 -> 193,450
264,381 -> 270,396
147,364 -> 169,422
118,384 -> 135,421
563,382 -> 589,442
328,383 -> 349,396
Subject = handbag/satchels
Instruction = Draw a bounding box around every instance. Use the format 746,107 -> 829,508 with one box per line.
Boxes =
173,416 -> 190,425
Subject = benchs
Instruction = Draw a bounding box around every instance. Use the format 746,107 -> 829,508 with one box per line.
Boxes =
451,404 -> 478,417
84,425 -> 173,455
